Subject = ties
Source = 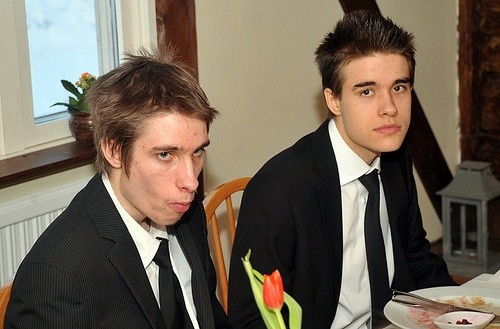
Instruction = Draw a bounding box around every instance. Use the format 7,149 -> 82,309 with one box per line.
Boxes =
153,237 -> 196,329
357,169 -> 395,329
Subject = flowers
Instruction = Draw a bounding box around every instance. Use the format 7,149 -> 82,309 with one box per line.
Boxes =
49,72 -> 97,112
241,249 -> 303,329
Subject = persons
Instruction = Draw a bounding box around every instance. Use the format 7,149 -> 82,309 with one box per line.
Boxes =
3,56 -> 229,329
228,10 -> 456,329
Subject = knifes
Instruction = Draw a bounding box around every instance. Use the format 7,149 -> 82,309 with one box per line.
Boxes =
393,289 -> 500,323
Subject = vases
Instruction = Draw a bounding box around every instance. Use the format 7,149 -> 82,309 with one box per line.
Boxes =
68,109 -> 96,147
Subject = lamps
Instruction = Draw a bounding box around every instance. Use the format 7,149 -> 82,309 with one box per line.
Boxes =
436,160 -> 500,277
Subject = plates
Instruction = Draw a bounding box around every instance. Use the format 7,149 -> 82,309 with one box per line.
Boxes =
384,286 -> 500,329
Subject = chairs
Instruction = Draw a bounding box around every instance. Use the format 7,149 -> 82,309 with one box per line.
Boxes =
202,178 -> 252,316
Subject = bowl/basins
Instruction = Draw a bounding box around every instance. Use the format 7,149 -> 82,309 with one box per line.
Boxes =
433,311 -> 495,329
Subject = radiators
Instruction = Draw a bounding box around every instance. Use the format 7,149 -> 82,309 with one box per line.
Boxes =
0,173 -> 97,290
